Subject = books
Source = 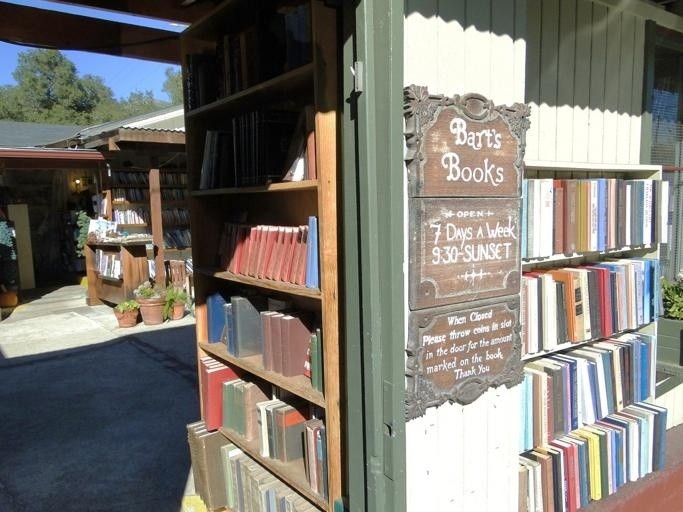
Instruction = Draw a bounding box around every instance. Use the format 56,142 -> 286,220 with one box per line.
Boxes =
517,179 -> 667,511
184,101 -> 326,511
93,171 -> 184,280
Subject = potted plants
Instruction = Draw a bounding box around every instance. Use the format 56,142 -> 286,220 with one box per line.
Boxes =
113,281 -> 187,328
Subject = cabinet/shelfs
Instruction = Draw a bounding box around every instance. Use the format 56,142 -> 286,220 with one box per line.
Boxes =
519,163 -> 662,512
178,0 -> 342,512
102,169 -> 192,291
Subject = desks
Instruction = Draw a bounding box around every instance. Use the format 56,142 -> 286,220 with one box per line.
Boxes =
86,240 -> 152,307
